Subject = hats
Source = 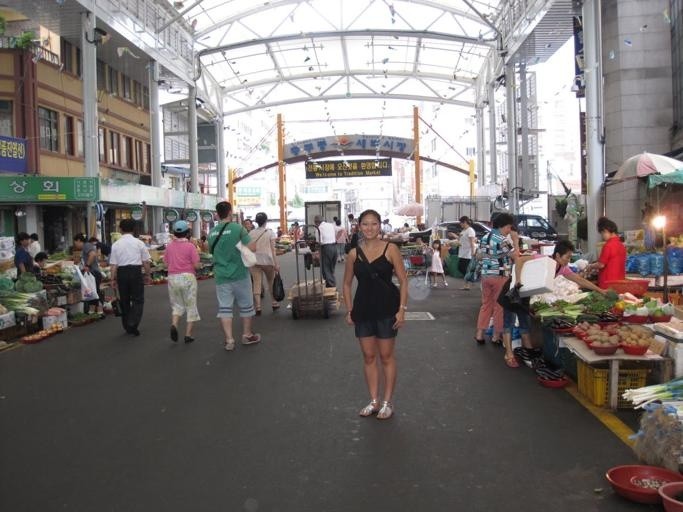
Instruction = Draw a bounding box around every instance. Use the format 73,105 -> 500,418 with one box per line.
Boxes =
173,221 -> 188,232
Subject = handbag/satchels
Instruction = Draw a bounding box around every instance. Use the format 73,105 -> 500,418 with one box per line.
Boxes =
111,300 -> 121,314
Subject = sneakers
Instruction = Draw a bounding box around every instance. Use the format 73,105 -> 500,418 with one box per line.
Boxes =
127,302 -> 279,350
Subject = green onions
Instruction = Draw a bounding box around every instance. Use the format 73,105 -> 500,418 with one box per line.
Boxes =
0,287 -> 40,310
621,374 -> 683,410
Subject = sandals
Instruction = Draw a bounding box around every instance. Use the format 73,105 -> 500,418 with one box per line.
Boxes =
376,401 -> 394,420
505,354 -> 518,367
360,399 -> 381,416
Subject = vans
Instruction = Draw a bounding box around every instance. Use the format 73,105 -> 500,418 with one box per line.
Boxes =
491,212 -> 558,241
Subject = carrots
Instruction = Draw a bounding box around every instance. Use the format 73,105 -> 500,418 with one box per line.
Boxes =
45,307 -> 65,316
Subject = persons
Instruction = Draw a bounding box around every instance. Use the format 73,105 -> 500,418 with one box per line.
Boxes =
109,218 -> 153,335
163,221 -> 205,339
245,212 -> 280,315
448,216 -> 477,290
313,212 -> 357,288
474,212 -> 520,344
427,239 -> 448,287
340,210 -> 408,418
382,219 -> 392,232
586,219 -> 625,294
497,240 -> 607,364
207,200 -> 262,352
402,223 -> 410,232
15,232 -> 48,281
74,234 -> 112,315
290,222 -> 301,238
277,227 -> 283,238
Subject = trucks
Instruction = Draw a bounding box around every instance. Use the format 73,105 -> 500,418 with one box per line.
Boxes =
303,200 -> 351,254
423,196 -> 514,229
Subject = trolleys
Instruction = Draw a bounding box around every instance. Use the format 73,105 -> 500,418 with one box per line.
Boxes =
292,223 -> 329,322
403,245 -> 436,289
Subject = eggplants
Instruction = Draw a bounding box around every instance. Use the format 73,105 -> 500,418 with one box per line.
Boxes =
36,273 -> 71,293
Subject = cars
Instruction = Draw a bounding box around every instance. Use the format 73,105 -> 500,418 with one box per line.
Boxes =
408,221 -> 491,244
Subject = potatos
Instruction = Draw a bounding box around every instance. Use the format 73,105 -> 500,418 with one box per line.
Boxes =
574,320 -> 653,349
21,324 -> 63,340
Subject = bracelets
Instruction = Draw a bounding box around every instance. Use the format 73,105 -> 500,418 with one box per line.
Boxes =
400,303 -> 408,311
346,310 -> 352,315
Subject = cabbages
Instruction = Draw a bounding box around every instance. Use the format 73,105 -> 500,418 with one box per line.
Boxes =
15,271 -> 43,294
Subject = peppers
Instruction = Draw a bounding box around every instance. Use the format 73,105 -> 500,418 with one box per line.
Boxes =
615,298 -> 665,318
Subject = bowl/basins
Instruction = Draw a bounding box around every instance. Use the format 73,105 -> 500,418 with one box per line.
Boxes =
621,313 -> 674,323
655,479 -> 682,512
604,464 -> 681,503
549,322 -> 650,355
536,375 -> 568,388
